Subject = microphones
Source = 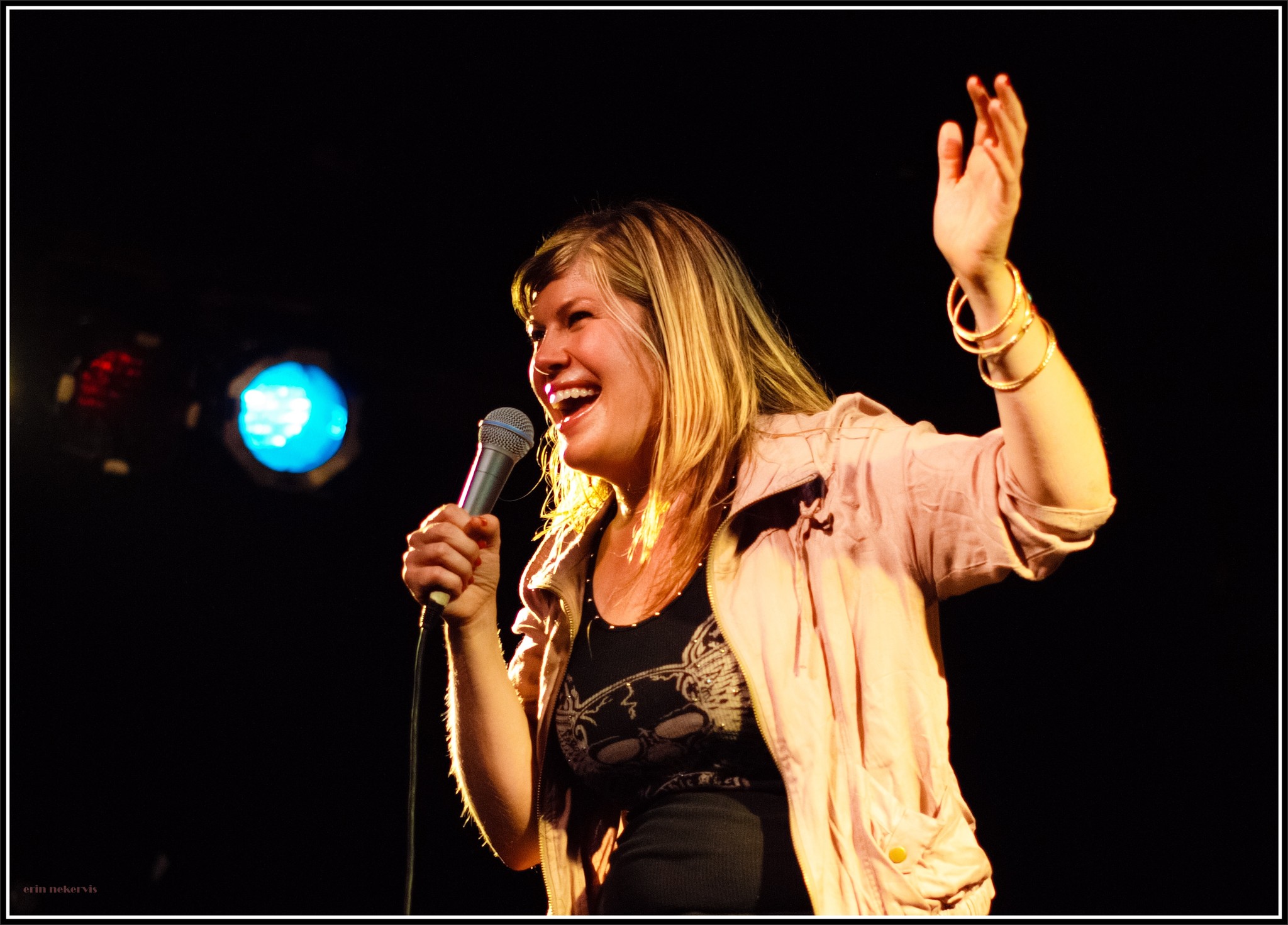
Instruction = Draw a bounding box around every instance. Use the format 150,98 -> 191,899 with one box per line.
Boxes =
419,407 -> 535,629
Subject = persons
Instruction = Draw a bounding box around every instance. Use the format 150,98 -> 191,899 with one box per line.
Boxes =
400,73 -> 1120,916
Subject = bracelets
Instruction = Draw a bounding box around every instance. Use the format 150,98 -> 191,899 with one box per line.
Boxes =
947,260 -> 1021,345
952,285 -> 1034,356
978,316 -> 1056,393
